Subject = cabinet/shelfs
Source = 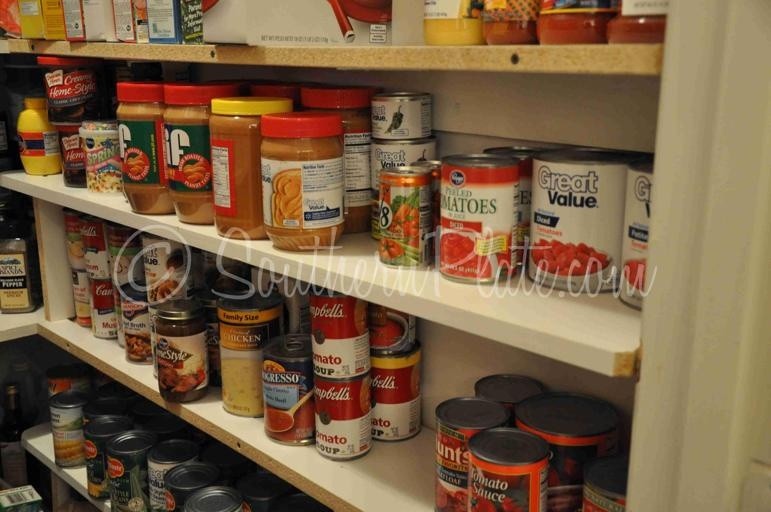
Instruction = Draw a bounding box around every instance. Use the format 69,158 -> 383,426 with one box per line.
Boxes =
0,33 -> 665,512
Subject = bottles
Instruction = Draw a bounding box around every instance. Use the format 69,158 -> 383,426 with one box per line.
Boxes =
1,218 -> 35,315
16,99 -> 63,177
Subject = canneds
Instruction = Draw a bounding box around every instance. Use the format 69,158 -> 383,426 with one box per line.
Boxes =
0,214 -> 41,314
45,79 -> 654,512
